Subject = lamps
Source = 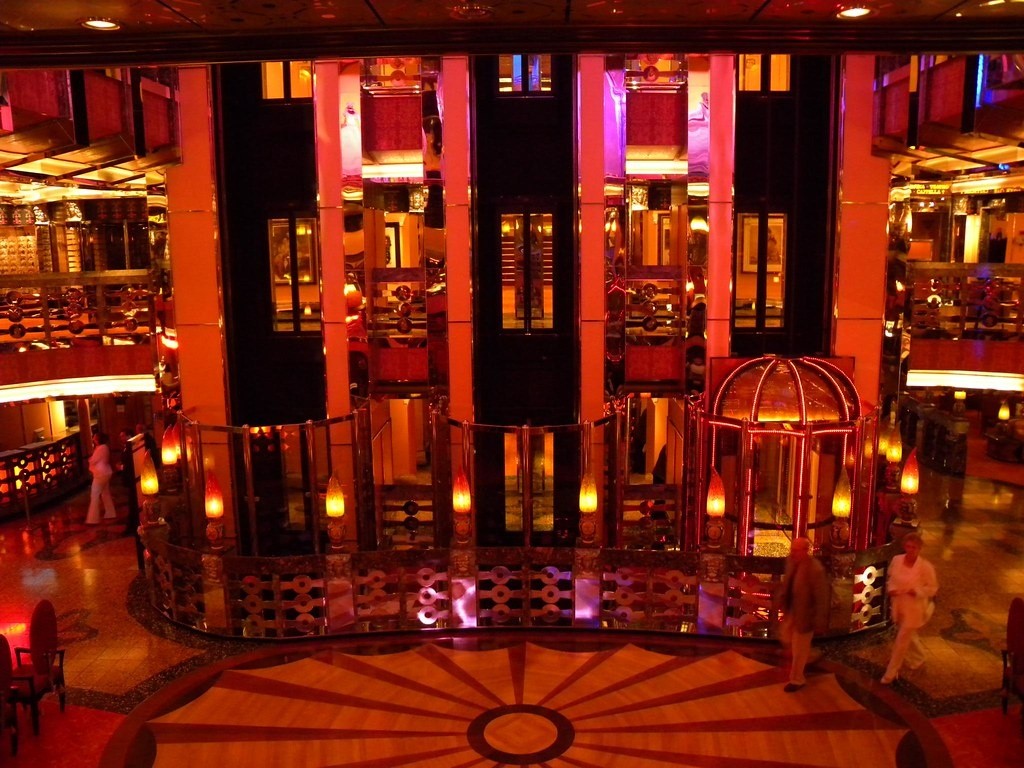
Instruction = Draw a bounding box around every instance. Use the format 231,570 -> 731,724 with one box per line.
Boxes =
326,475 -> 345,550
204,468 -> 224,551
161,423 -> 188,489
998,403 -> 1011,434
141,449 -> 159,524
707,468 -> 725,550
886,422 -> 920,525
579,469 -> 597,545
452,471 -> 471,546
832,463 -> 852,551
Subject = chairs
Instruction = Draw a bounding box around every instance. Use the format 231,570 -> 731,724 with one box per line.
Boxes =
1002,598 -> 1024,724
0,600 -> 64,756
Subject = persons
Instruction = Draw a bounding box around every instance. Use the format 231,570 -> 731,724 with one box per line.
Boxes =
85,423 -> 158,526
773,536 -> 828,693
650,444 -> 668,519
881,533 -> 937,683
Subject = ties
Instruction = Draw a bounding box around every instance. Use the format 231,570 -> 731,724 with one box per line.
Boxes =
785,565 -> 798,610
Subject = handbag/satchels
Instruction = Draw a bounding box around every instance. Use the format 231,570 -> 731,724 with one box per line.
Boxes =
924,601 -> 935,621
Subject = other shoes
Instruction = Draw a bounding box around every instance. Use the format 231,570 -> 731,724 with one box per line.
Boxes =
910,657 -> 927,670
783,683 -> 805,693
881,671 -> 899,684
